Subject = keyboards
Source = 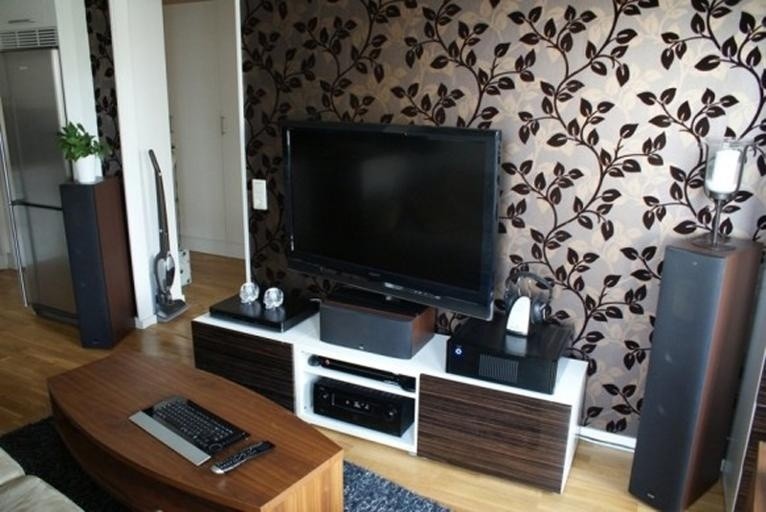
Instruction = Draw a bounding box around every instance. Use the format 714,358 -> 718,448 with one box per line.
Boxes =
128,395 -> 250,467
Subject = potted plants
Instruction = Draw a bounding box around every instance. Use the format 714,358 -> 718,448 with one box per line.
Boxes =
55,122 -> 104,185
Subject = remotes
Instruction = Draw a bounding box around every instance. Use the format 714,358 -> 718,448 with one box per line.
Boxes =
210,441 -> 275,475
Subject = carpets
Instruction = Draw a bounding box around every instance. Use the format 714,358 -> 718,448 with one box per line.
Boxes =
1,415 -> 455,512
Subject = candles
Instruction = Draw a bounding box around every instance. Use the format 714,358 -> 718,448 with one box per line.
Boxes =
710,150 -> 740,193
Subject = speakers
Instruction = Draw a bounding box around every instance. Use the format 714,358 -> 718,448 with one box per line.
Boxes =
628,231 -> 765,512
59,176 -> 136,350
320,298 -> 437,359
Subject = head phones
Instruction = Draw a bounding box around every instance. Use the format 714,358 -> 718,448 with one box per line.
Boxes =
503,271 -> 553,325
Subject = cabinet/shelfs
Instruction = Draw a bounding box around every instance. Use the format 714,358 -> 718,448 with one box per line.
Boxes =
191,298 -> 589,494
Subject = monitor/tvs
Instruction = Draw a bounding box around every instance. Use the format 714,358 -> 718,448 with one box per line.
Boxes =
280,118 -> 502,323
